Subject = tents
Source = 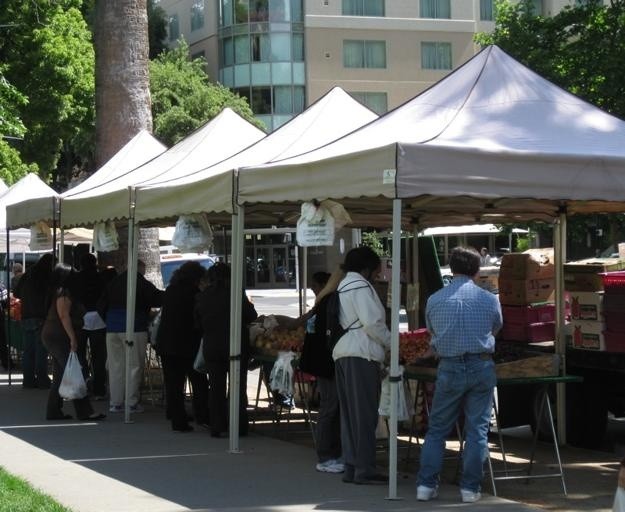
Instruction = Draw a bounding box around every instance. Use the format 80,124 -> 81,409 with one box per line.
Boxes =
4,127 -> 167,390
57,106 -> 268,424
125,84 -> 380,457
235,40 -> 623,504
0,170 -> 59,249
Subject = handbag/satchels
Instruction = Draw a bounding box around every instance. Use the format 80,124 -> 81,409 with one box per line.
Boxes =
299,331 -> 336,382
147,306 -> 163,346
193,337 -> 209,374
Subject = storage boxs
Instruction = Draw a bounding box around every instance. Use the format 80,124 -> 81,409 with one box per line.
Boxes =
497,280 -> 555,305
569,293 -> 603,322
571,323 -> 603,351
601,313 -> 625,333
598,293 -> 625,312
501,322 -> 555,342
597,272 -> 625,292
501,306 -> 555,325
565,273 -> 604,291
498,254 -> 555,280
603,333 -> 625,353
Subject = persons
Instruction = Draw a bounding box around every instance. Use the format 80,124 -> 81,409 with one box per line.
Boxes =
105,260 -> 167,414
13,252 -> 59,388
42,271 -> 108,422
330,246 -> 391,485
196,261 -> 258,440
154,260 -> 210,433
11,262 -> 24,294
416,246 -> 504,503
66,253 -> 106,402
308,273 -> 346,473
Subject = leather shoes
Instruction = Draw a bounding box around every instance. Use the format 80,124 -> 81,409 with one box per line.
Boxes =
196,420 -> 218,432
172,423 -> 195,433
187,413 -> 195,423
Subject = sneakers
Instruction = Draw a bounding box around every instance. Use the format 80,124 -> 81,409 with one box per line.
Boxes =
459,488 -> 483,505
416,482 -> 441,502
129,404 -> 145,415
38,377 -> 52,389
354,467 -> 388,486
342,468 -> 354,482
23,375 -> 36,389
315,459 -> 346,474
48,413 -> 74,421
109,404 -> 125,414
94,395 -> 105,403
79,414 -> 107,422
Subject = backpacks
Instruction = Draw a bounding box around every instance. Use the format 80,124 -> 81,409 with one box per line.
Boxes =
314,279 -> 374,354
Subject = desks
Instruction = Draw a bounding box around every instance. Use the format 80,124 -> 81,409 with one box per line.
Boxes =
144,325 -> 163,408
4,316 -> 24,348
242,348 -> 581,498
